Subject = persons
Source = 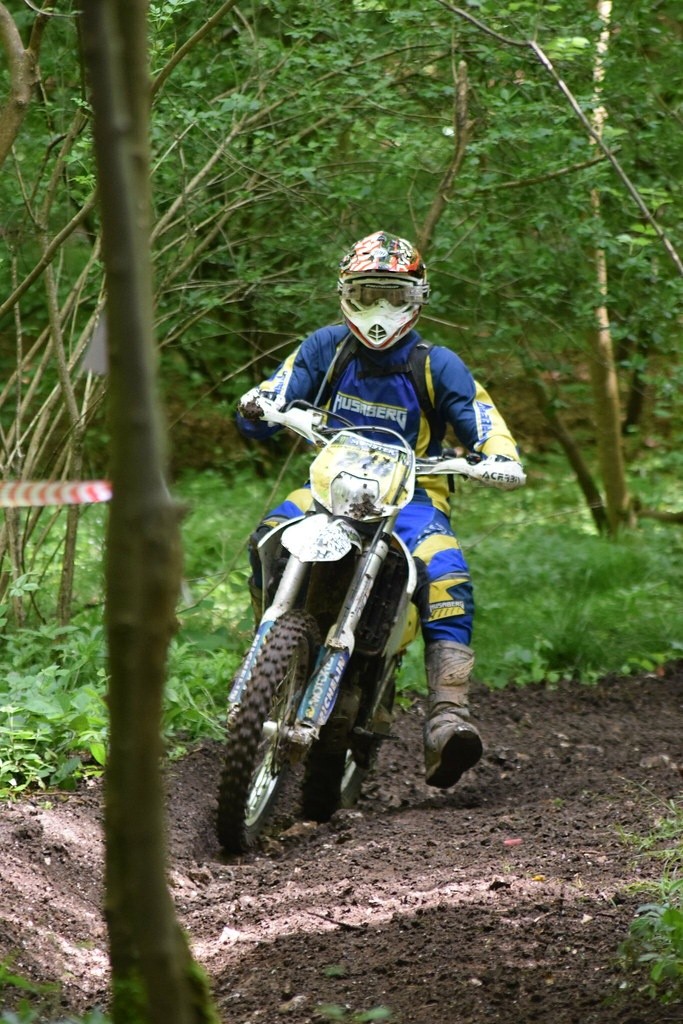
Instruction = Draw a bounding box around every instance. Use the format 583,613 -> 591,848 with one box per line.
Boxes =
237,226 -> 525,789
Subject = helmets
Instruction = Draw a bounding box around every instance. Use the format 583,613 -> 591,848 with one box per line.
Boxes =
337,231 -> 429,351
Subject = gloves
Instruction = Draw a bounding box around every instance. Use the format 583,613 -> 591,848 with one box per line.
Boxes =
476,456 -> 524,492
238,389 -> 266,419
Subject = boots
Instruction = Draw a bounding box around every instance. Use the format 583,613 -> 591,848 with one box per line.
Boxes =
423,640 -> 483,789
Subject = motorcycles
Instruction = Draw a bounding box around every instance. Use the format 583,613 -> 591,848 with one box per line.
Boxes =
211,388 -> 528,855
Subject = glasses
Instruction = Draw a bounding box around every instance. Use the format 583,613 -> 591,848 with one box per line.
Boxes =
337,278 -> 428,304
